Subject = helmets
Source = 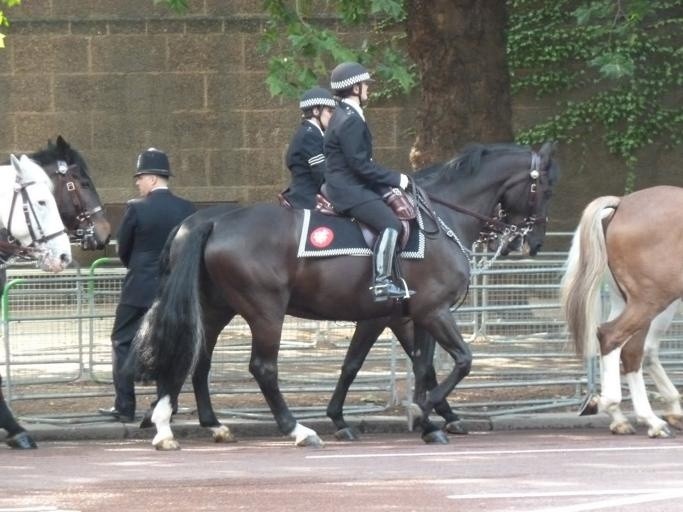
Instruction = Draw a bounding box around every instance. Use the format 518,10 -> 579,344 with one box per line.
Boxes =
132,147 -> 172,178
328,61 -> 372,91
298,89 -> 334,112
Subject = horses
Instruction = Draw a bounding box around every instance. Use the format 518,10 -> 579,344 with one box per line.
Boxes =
121,132 -> 557,445
1,134 -> 113,450
558,185 -> 683,439
0,150 -> 72,272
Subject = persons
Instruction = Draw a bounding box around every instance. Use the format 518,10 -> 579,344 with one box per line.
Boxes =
98,147 -> 196,422
320,60 -> 418,303
277,86 -> 337,209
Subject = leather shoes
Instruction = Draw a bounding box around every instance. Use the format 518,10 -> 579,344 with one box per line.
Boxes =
98,407 -> 132,424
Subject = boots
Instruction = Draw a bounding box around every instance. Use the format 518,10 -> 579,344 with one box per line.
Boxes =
368,226 -> 416,303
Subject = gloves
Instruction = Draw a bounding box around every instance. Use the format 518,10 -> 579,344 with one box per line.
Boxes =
400,174 -> 410,191
